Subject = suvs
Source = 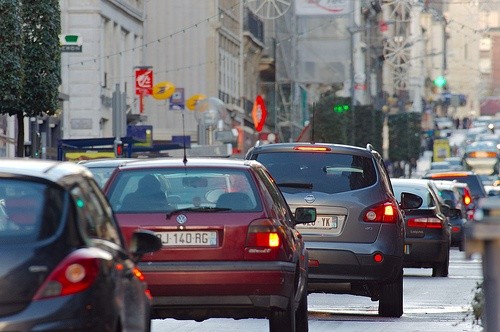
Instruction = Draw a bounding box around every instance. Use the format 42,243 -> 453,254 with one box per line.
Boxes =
243,140 -> 423,318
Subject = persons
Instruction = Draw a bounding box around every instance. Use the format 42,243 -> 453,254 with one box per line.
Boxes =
135,174 -> 162,202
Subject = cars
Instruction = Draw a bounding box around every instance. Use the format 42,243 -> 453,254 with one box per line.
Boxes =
0,158 -> 152,332
102,158 -> 317,332
77,159 -> 144,191
390,116 -> 500,277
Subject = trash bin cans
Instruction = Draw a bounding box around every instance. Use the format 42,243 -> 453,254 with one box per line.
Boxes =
472,219 -> 500,332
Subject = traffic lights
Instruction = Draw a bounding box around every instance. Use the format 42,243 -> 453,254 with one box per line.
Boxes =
434,77 -> 446,86
